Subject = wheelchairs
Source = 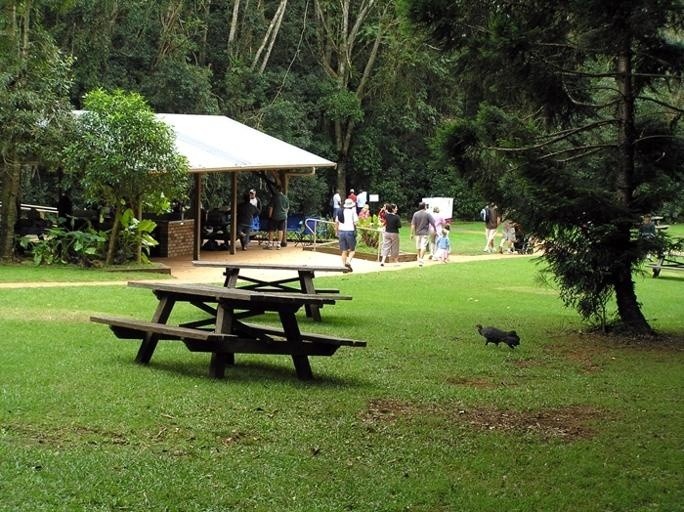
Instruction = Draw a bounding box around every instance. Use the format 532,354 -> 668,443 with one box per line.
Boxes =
508,236 -> 533,255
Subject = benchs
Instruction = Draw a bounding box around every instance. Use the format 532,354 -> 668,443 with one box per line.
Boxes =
90,283 -> 365,379
631,213 -> 684,276
201,222 -> 244,251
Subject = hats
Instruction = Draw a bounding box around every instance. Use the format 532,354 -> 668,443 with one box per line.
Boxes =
343,198 -> 356,208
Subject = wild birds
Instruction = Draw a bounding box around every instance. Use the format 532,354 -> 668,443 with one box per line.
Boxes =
475,322 -> 520,350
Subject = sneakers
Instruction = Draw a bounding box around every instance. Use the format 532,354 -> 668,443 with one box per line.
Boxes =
263,241 -> 287,250
484,246 -> 513,255
380,262 -> 384,266
418,254 -> 448,266
345,262 -> 353,272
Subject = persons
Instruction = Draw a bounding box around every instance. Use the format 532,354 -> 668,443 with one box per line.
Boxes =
200,201 -> 207,246
236,193 -> 260,249
248,188 -> 262,230
639,215 -> 657,239
173,201 -> 180,213
263,185 -> 290,250
481,201 -> 538,255
207,202 -> 229,245
410,203 -> 450,267
331,188 -> 401,273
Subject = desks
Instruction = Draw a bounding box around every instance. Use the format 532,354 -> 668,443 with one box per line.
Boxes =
127,257 -> 350,381
